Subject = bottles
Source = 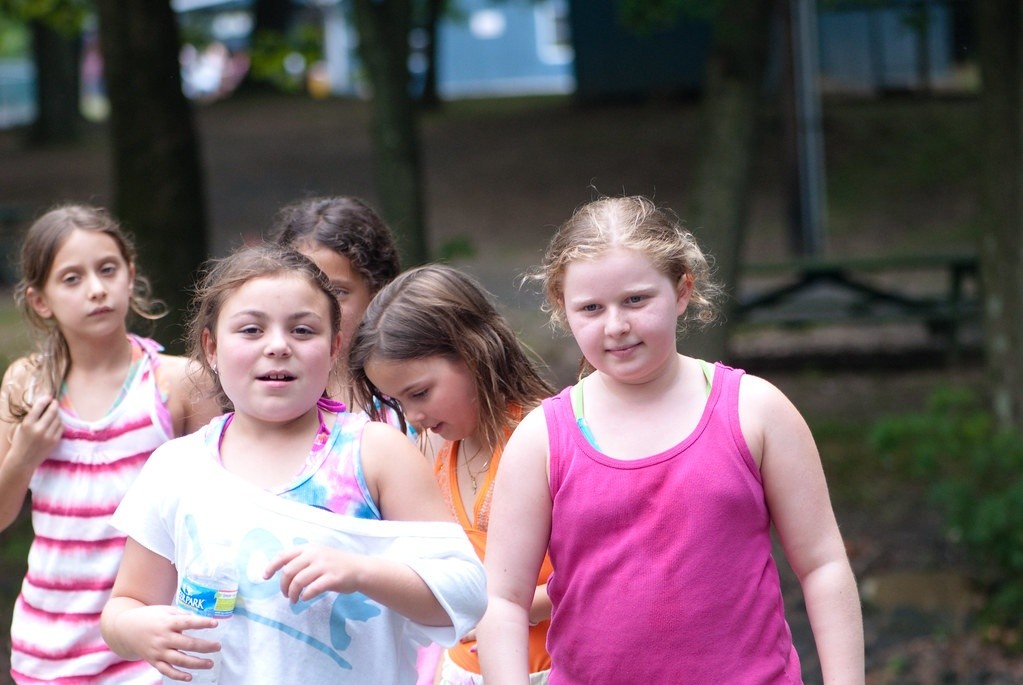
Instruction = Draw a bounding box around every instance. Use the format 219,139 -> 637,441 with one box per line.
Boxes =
161,531 -> 238,685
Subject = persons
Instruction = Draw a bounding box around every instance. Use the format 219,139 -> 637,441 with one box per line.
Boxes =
0,201 -> 223,685
268,194 -> 419,443
99,239 -> 491,685
475,195 -> 866,685
344,263 -> 562,685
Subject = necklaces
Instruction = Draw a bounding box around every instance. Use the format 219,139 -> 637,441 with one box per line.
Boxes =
462,438 -> 495,489
